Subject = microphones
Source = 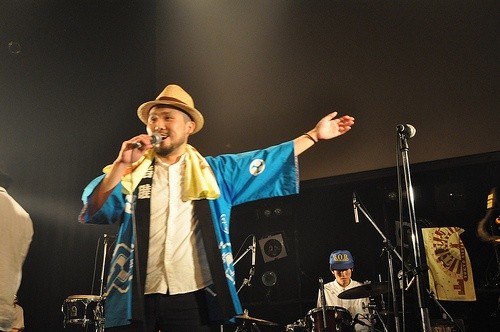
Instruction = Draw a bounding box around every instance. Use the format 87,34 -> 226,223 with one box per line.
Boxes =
133,132 -> 162,149
252,237 -> 257,266
352,195 -> 359,223
397,123 -> 417,139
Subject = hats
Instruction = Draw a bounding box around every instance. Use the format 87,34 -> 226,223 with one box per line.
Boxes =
329,250 -> 354,270
137,84 -> 204,135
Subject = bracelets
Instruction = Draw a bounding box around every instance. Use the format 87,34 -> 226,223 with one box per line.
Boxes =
303,133 -> 317,144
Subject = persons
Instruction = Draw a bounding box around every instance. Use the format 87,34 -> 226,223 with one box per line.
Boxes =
0,187 -> 35,332
78,84 -> 354,332
316,249 -> 373,332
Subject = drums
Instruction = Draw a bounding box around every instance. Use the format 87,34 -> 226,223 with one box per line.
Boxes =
306,306 -> 356,332
286,324 -> 306,332
64,296 -> 105,325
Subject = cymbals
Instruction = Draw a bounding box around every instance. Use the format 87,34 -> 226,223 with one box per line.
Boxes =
235,315 -> 277,325
338,281 -> 398,299
